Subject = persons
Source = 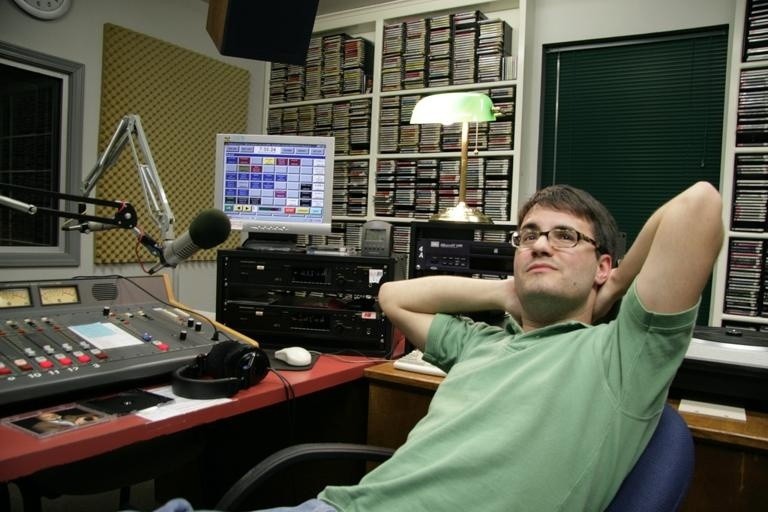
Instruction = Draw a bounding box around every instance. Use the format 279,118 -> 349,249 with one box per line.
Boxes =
139,177 -> 727,512
30,411 -> 102,434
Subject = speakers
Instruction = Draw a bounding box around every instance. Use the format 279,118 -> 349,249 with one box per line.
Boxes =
206,0 -> 320,66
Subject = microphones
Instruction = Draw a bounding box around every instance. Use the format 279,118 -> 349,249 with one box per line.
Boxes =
149,209 -> 231,276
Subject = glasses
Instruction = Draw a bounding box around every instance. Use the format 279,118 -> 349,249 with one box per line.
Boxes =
510,228 -> 609,253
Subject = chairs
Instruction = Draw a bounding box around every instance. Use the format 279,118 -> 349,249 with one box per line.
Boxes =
223,401 -> 701,510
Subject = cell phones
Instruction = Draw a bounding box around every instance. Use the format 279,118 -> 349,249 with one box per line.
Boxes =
727,326 -> 743,335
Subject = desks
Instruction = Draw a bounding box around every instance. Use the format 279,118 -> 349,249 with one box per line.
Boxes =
1,344 -> 388,479
364,356 -> 767,510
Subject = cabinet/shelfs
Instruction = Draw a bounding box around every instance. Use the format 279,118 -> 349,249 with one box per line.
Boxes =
707,3 -> 768,331
260,1 -> 538,259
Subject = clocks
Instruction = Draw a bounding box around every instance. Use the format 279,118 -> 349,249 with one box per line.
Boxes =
14,0 -> 73,22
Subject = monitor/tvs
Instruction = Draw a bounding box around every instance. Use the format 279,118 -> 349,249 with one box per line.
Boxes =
214,134 -> 335,250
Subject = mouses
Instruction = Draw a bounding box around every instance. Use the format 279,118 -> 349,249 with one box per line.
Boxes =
275,346 -> 312,366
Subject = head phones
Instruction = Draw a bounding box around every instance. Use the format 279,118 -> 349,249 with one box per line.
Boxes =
174,340 -> 270,399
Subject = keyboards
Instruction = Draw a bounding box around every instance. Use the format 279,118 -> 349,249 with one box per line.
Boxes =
393,348 -> 447,377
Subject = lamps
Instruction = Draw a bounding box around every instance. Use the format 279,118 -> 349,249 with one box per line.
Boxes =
389,90 -> 515,224
